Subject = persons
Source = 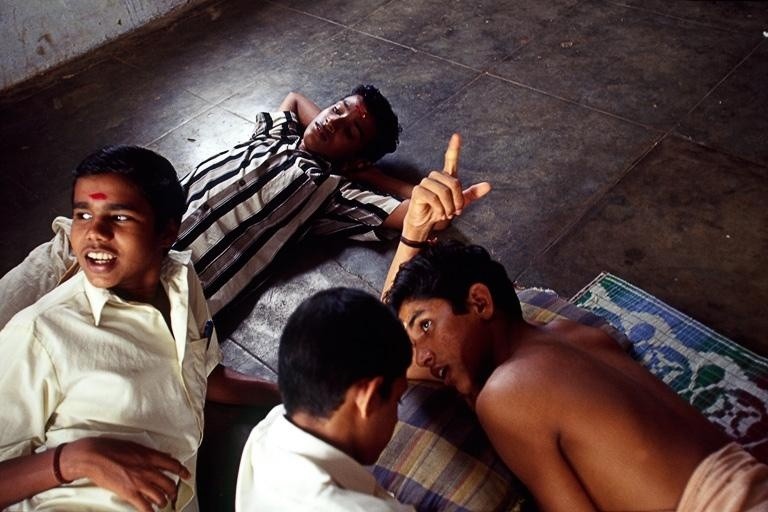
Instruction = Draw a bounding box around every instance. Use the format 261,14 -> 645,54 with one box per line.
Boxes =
379,134 -> 767,510
233,288 -> 422,511
1,143 -> 282,512
0,84 -> 450,329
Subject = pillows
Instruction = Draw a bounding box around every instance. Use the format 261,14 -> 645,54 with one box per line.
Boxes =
372,282 -> 634,511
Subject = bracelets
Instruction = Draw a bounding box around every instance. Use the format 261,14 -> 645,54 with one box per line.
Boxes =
52,441 -> 72,485
398,234 -> 425,250
